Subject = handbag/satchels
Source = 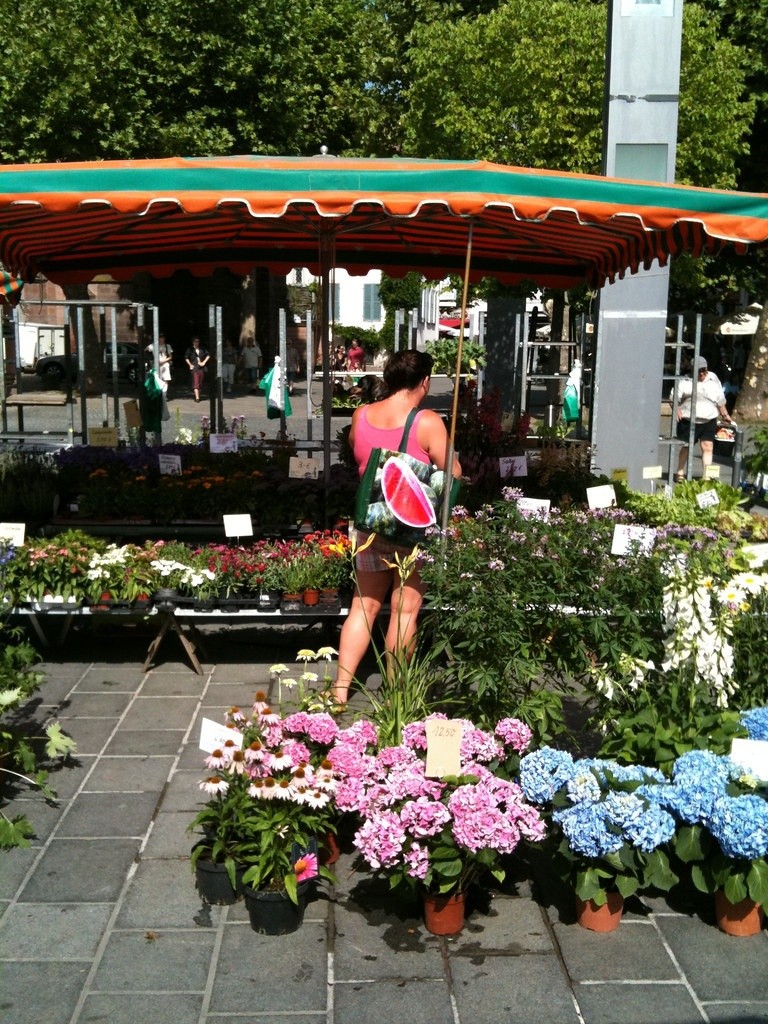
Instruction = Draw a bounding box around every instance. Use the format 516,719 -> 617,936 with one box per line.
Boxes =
353,407 -> 464,555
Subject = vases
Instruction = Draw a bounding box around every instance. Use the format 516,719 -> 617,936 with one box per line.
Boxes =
2,583 -> 342,612
716,891 -> 762,938
576,887 -> 622,933
421,888 -> 464,937
188,829 -> 339,936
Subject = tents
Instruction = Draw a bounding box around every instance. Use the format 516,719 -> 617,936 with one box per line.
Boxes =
0,144 -> 768,468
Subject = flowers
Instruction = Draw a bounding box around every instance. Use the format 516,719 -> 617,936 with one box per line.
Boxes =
2,529 -> 352,607
524,750 -> 678,906
670,749 -> 768,917
739,707 -> 768,741
174,428 -> 195,448
199,414 -> 248,445
191,647 -> 339,905
329,711 -> 544,896
445,378 -> 531,451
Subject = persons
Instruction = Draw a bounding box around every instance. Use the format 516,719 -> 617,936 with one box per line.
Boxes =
184,336 -> 212,402
670,354 -> 741,483
214,339 -> 240,393
286,339 -> 300,394
239,337 -> 263,395
328,349 -> 462,705
143,332 -> 175,403
330,343 -> 348,371
346,338 -> 367,387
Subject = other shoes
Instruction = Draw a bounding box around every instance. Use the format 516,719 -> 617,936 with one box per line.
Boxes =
676,473 -> 684,484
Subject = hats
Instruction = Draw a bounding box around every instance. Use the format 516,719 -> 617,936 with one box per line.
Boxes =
691,357 -> 707,374
